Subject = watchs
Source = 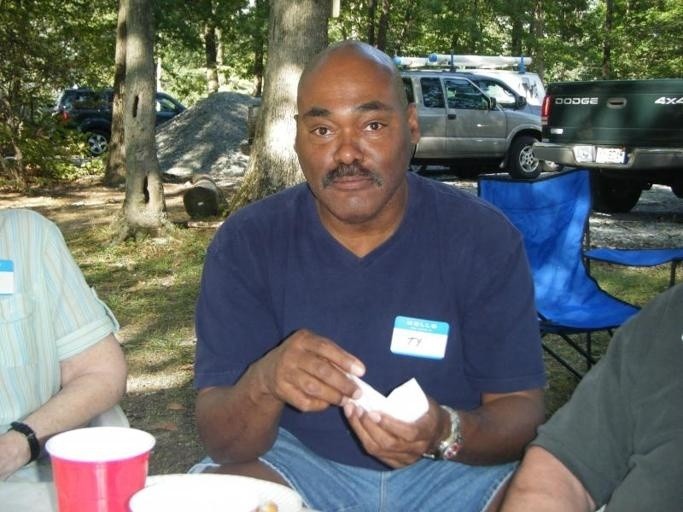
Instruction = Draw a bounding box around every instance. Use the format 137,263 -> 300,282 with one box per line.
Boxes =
420,399 -> 466,465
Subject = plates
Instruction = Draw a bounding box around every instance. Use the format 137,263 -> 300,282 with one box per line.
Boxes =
126,474 -> 303,512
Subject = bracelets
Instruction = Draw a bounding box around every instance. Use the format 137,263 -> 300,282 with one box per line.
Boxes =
8,420 -> 43,468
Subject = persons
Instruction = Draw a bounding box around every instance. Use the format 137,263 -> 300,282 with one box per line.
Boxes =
501,279 -> 683,511
0,207 -> 135,484
184,41 -> 548,512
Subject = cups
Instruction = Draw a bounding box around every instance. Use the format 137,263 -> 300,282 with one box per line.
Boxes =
42,428 -> 153,512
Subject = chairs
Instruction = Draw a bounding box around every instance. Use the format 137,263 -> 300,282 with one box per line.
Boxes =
475,164 -> 644,393
581,204 -> 683,295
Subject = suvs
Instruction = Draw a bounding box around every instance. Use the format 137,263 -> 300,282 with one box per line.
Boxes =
50,88 -> 191,155
